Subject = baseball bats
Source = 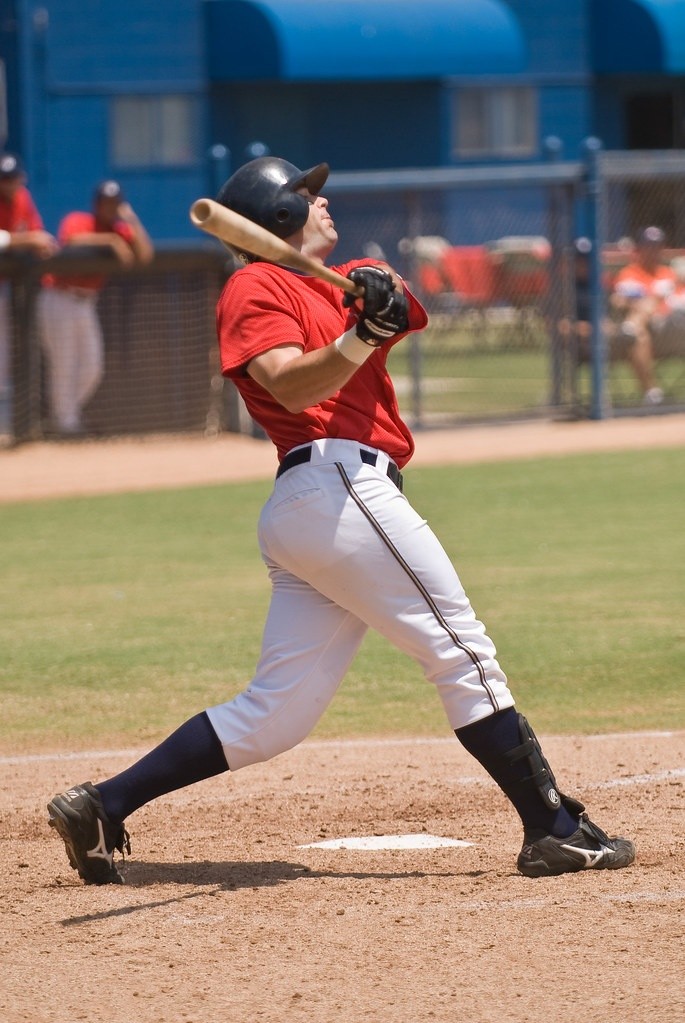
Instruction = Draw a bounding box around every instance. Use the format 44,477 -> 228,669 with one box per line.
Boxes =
189,198 -> 365,296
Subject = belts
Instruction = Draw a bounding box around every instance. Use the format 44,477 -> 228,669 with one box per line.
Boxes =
276,446 -> 404,495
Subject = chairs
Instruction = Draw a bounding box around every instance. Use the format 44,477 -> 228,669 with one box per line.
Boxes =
413,233 -> 685,418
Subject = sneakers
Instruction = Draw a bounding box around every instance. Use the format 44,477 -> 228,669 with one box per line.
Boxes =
518,815 -> 639,879
49,782 -> 128,886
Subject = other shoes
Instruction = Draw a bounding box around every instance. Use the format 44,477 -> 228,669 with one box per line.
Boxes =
614,323 -> 636,349
640,388 -> 665,406
57,422 -> 95,444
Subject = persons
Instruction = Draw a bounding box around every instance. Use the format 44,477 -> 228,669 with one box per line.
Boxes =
48,158 -> 635,886
609,223 -> 685,405
35,181 -> 154,435
0,151 -> 60,450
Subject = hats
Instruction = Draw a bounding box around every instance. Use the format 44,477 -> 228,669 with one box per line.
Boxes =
574,237 -> 593,257
639,225 -> 668,246
1,152 -> 22,176
94,180 -> 123,199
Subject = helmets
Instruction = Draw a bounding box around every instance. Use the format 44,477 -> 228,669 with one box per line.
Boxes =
217,155 -> 331,239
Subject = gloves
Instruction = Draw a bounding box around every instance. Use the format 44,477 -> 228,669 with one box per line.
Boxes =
339,269 -> 413,350
